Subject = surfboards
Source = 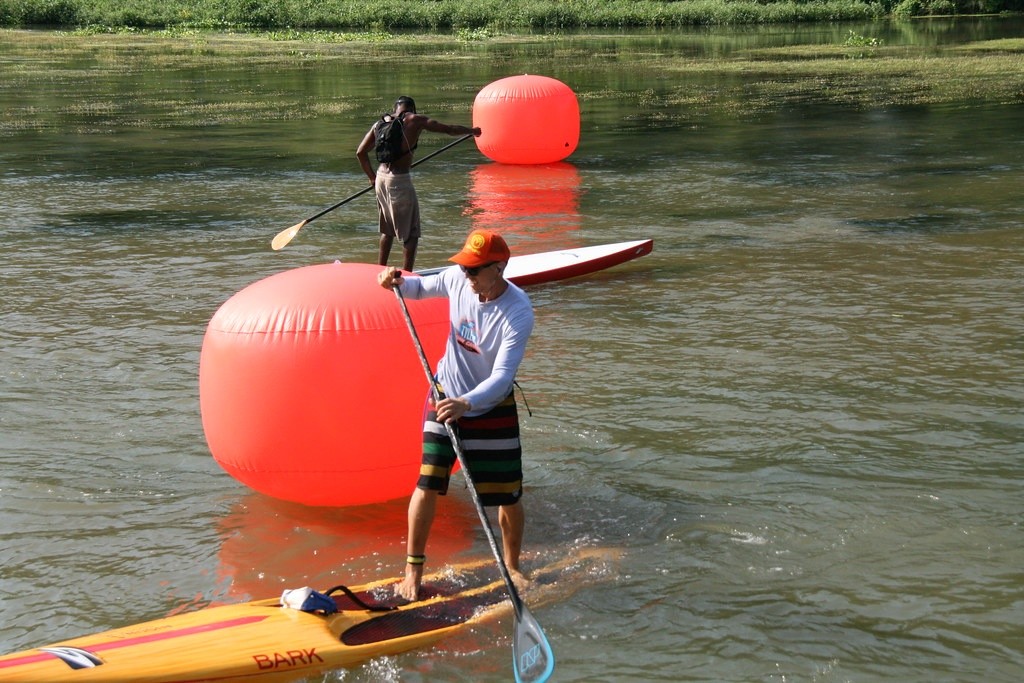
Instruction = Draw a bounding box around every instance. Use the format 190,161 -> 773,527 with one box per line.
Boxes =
0,538 -> 618,683
410,238 -> 655,287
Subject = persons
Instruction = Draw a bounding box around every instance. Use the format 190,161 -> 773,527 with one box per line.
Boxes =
356,96 -> 482,273
379,228 -> 536,601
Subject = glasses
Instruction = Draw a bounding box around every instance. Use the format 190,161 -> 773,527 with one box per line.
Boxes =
459,261 -> 497,276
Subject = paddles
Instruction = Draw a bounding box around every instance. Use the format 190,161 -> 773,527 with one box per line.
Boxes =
270,130 -> 474,252
388,282 -> 554,683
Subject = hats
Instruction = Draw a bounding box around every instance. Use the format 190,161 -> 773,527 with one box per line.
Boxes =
448,230 -> 510,266
